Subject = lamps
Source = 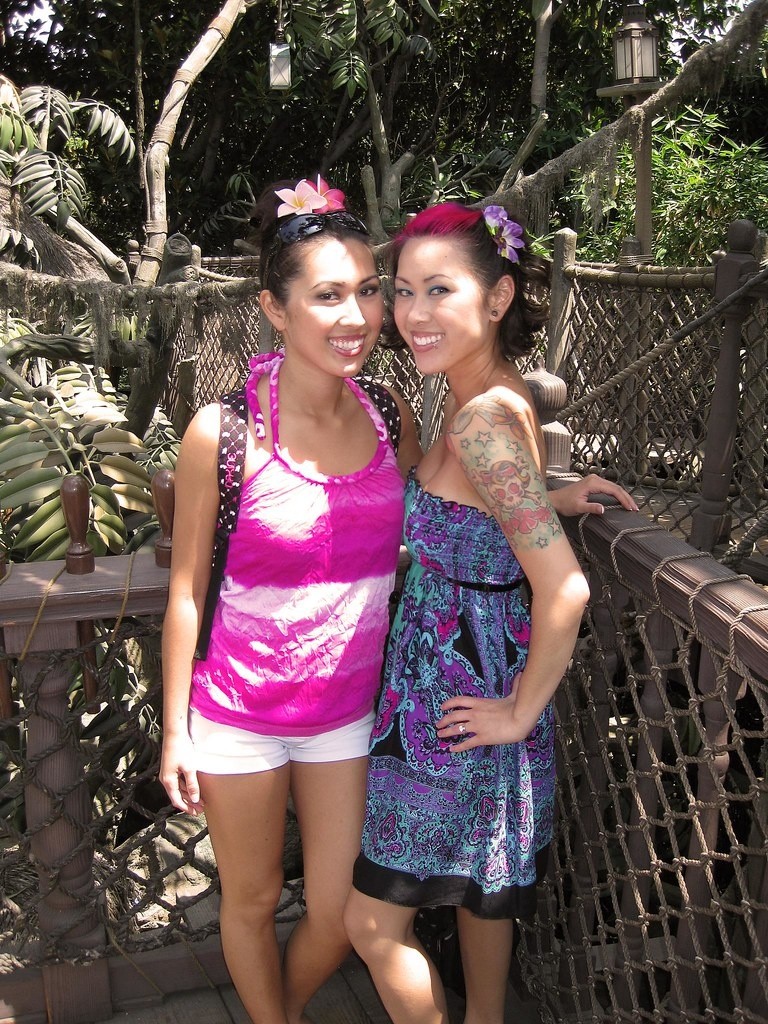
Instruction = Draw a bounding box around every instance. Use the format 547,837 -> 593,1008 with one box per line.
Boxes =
612,3 -> 658,84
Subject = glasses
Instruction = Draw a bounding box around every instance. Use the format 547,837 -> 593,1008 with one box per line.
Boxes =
262,211 -> 369,288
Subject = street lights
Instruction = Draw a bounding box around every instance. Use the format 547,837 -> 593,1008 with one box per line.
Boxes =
608,3 -> 661,475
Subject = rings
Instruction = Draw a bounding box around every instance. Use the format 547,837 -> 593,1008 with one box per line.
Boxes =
458,723 -> 466,735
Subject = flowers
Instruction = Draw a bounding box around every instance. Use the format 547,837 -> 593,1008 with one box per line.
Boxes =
484,205 -> 524,265
274,173 -> 346,218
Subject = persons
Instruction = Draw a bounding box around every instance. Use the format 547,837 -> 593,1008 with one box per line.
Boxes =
342,204 -> 590,1024
159,175 -> 639,1024
